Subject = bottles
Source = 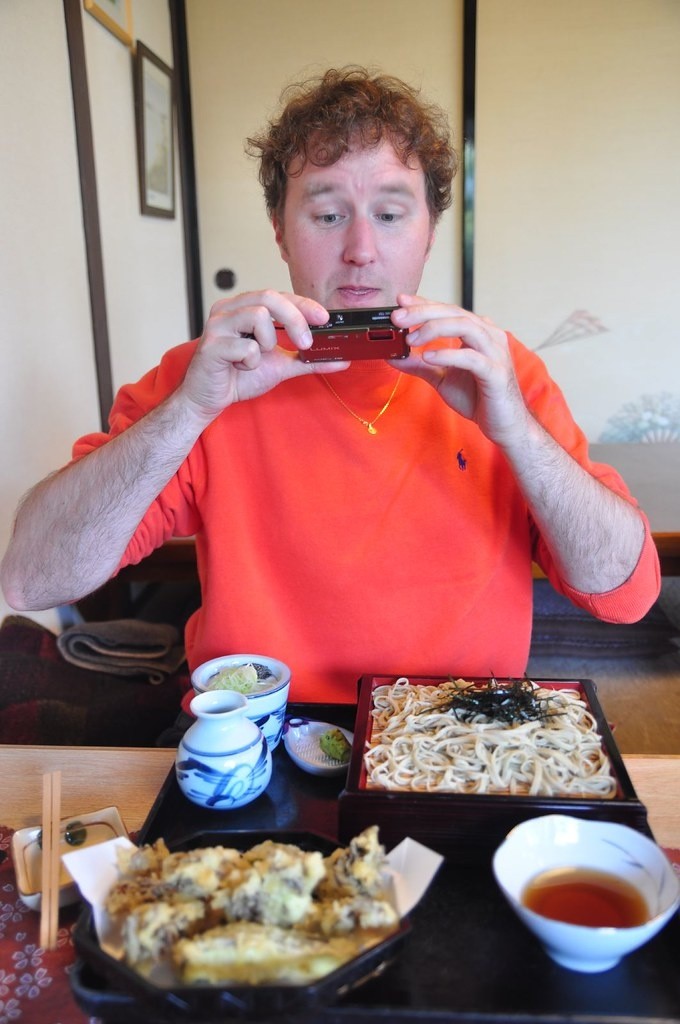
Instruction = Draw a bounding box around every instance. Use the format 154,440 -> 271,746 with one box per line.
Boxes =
174,690 -> 272,810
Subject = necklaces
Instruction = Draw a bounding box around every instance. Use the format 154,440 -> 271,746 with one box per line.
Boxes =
321,370 -> 404,434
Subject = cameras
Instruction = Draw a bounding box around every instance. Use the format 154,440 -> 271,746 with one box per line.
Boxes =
298,305 -> 410,363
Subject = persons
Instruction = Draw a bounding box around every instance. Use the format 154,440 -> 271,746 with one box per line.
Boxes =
0,66 -> 662,748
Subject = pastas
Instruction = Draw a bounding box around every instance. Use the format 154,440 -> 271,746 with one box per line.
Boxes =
367,675 -> 618,800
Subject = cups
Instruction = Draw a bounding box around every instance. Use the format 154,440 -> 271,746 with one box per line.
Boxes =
191,654 -> 292,752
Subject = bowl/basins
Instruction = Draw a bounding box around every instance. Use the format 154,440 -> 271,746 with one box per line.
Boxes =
492,816 -> 680,975
336,673 -> 646,840
72,831 -> 413,1021
12,806 -> 133,913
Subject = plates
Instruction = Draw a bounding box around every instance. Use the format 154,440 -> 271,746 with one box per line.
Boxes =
280,716 -> 354,775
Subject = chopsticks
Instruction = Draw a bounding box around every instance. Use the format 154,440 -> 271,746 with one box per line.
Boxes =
41,770 -> 63,952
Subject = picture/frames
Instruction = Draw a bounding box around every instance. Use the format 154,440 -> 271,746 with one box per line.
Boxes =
83,0 -> 135,48
134,40 -> 177,221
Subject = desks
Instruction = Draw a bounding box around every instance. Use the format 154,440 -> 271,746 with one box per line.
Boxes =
1,740 -> 680,1023
